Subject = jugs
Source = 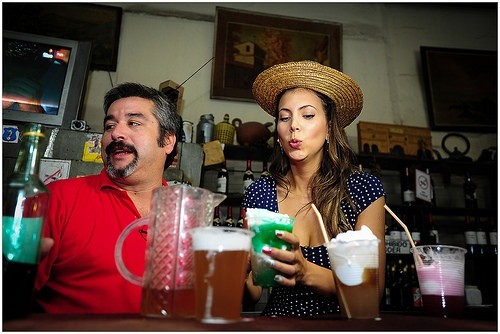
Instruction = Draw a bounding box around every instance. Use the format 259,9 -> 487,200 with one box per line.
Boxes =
114,185 -> 227,319
233,116 -> 274,145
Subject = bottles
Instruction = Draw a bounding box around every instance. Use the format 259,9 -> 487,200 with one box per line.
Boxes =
385,219 -> 498,256
261,159 -> 269,177
219,157 -> 229,195
244,159 -> 253,195
401,165 -> 416,209
213,113 -> 236,143
2,121 -> 48,320
426,167 -> 436,210
214,204 -> 244,227
382,260 -> 422,311
465,176 -> 477,209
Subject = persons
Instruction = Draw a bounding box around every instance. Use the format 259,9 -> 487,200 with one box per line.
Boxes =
24,81 -> 193,315
240,60 -> 387,319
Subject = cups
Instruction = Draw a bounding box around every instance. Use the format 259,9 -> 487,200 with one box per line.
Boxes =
243,216 -> 294,287
182,121 -> 193,144
187,225 -> 254,324
322,238 -> 381,319
409,242 -> 468,319
465,286 -> 481,307
197,115 -> 214,143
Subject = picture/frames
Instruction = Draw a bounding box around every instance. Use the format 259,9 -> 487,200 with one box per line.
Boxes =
419,45 -> 498,135
209,6 -> 345,103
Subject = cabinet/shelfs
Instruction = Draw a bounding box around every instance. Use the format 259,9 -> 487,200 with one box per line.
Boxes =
204,141 -> 497,306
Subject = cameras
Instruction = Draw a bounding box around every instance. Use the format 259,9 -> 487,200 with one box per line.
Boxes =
71,119 -> 86,132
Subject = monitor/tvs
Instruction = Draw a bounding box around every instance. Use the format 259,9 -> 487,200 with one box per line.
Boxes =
419,45 -> 497,133
3,30 -> 79,128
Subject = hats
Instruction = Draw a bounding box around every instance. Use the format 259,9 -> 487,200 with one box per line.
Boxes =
251,60 -> 365,131
